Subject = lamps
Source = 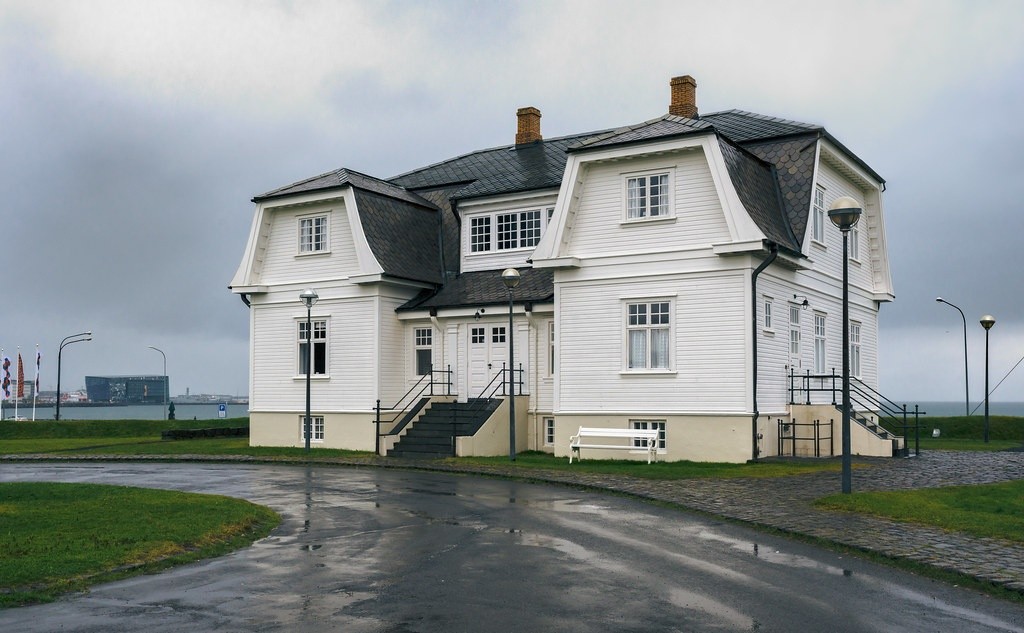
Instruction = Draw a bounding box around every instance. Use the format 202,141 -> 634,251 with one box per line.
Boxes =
793,294 -> 811,311
473,308 -> 485,322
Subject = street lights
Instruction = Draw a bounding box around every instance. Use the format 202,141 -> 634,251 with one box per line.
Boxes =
299,287 -> 319,453
148,346 -> 167,420
979,314 -> 996,443
936,297 -> 970,417
826,196 -> 863,494
55,332 -> 92,420
502,268 -> 522,462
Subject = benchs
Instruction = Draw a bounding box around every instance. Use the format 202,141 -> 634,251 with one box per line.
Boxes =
569,425 -> 660,464
